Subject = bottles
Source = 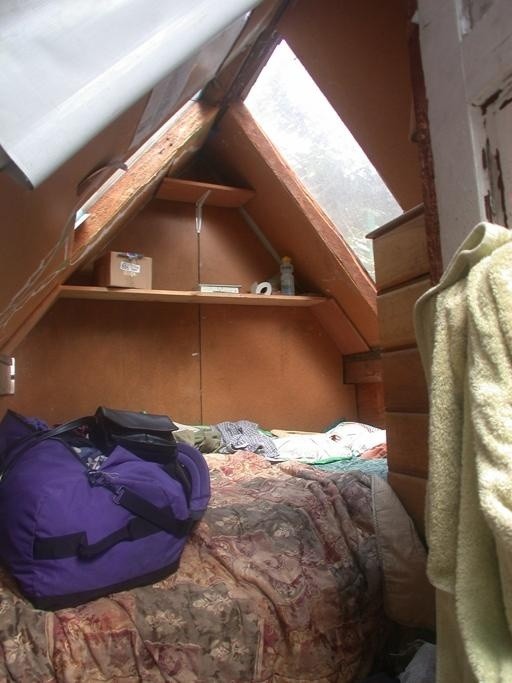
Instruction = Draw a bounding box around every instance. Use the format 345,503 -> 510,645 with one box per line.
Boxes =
280,256 -> 295,295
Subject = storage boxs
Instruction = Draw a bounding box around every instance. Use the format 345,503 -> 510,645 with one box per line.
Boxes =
93,250 -> 152,290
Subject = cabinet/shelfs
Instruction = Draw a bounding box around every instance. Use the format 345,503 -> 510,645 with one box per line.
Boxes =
365,200 -> 430,549
61,177 -> 329,306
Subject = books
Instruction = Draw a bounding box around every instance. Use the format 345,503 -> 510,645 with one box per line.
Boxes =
193,283 -> 242,294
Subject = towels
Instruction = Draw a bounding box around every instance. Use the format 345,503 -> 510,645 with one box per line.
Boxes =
413,221 -> 511,683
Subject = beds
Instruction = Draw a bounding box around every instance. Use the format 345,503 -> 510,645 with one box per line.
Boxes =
0,420 -> 437,683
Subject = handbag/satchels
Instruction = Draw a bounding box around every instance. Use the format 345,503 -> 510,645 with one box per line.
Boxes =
92,404 -> 179,462
0,407 -> 210,611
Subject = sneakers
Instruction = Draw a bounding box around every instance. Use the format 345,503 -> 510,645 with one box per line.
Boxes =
388,641 -> 423,673
